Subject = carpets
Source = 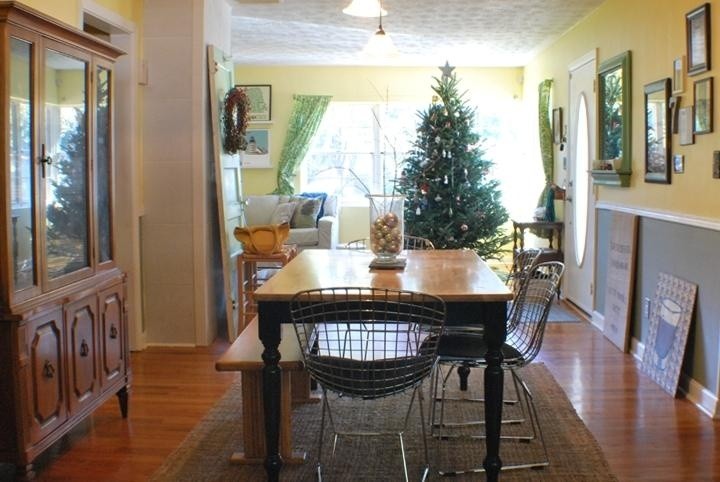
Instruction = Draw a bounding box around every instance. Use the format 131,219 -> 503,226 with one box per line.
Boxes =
144,357 -> 619,480
503,292 -> 582,325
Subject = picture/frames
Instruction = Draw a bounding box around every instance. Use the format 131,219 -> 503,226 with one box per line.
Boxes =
671,152 -> 685,175
682,3 -> 712,77
641,76 -> 674,186
234,83 -> 272,121
710,149 -> 720,181
669,55 -> 687,99
238,126 -> 271,166
692,75 -> 715,136
550,106 -> 564,146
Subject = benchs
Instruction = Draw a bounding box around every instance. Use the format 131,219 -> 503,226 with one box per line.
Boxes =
215,307 -> 325,468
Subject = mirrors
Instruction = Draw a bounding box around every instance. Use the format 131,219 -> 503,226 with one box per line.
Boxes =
585,51 -> 633,189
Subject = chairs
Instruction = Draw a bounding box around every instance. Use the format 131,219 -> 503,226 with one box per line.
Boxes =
423,243 -> 549,429
286,281 -> 448,482
419,261 -> 570,478
345,236 -> 446,429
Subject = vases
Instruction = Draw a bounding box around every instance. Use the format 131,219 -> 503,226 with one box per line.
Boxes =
366,192 -> 411,264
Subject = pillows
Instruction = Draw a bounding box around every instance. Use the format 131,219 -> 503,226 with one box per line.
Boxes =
266,190 -> 328,231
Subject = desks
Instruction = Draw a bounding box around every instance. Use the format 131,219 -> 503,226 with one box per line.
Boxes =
508,216 -> 565,305
235,243 -> 298,339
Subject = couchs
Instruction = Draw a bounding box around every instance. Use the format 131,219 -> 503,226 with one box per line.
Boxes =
240,193 -> 341,269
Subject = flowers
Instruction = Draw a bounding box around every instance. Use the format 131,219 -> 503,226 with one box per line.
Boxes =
220,85 -> 256,156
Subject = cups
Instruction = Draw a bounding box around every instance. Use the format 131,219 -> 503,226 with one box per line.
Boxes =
651,297 -> 681,372
364,195 -> 408,263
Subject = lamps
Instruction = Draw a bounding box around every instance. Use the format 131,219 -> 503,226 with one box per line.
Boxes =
354,0 -> 402,64
340,0 -> 389,22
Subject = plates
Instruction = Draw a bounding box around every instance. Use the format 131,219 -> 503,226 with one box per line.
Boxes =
368,258 -> 409,270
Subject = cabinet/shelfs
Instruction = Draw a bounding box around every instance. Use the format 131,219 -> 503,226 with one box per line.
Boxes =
0,0 -> 133,481
233,120 -> 275,170
